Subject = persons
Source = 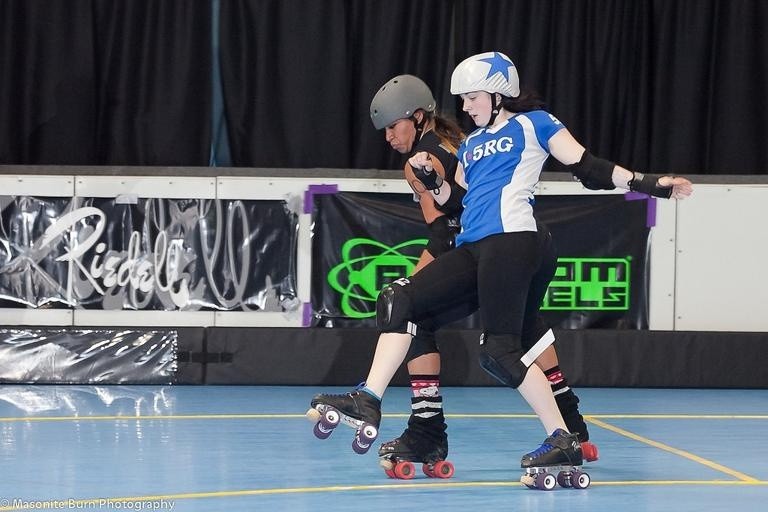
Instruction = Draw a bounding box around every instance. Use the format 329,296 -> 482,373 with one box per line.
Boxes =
370,74 -> 589,464
310,51 -> 693,468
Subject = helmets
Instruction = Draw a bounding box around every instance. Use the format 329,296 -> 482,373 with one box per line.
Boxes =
450,51 -> 522,100
370,74 -> 439,130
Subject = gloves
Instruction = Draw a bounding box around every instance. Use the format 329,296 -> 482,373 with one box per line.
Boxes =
629,169 -> 676,200
408,150 -> 443,191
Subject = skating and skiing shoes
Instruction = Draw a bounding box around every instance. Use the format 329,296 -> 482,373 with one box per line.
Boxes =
519,411 -> 599,490
305,385 -> 381,454
378,411 -> 456,481
